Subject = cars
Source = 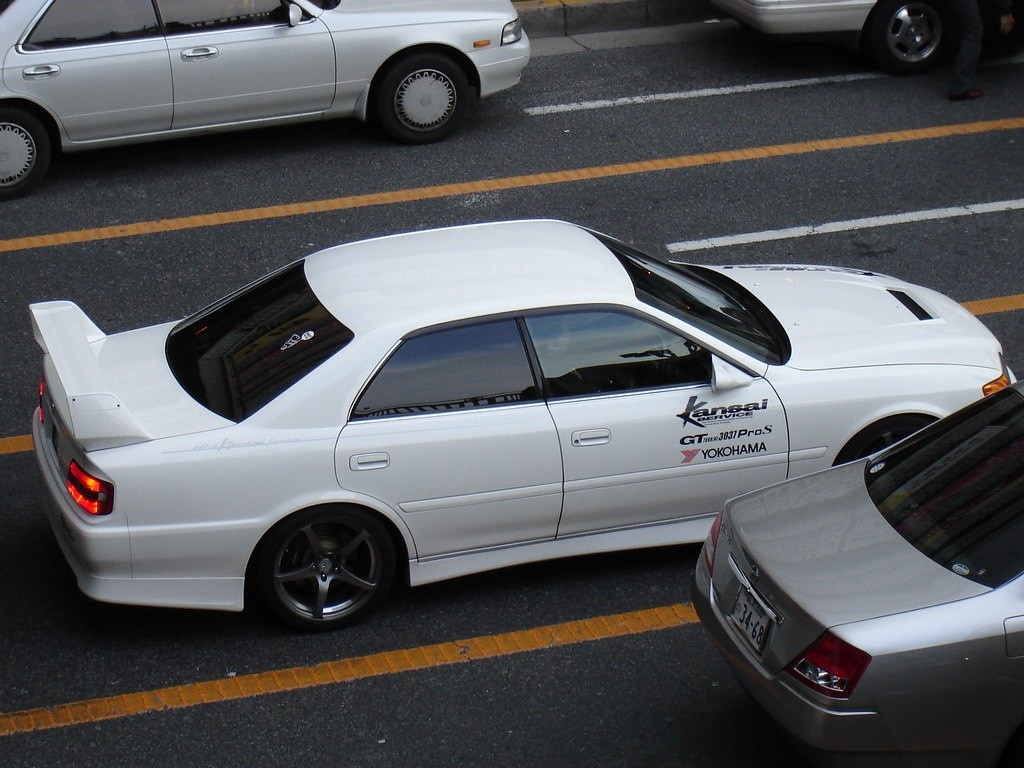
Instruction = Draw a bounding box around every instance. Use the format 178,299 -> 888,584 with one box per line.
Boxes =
688,381 -> 1023,768
719,1 -> 1024,78
27,219 -> 1022,637
0,0 -> 530,203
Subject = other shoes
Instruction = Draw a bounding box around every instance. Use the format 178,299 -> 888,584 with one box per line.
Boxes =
948,89 -> 983,101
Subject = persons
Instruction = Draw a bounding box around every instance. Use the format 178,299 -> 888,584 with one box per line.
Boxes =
948,0 -> 1015,101
539,315 -> 594,396
